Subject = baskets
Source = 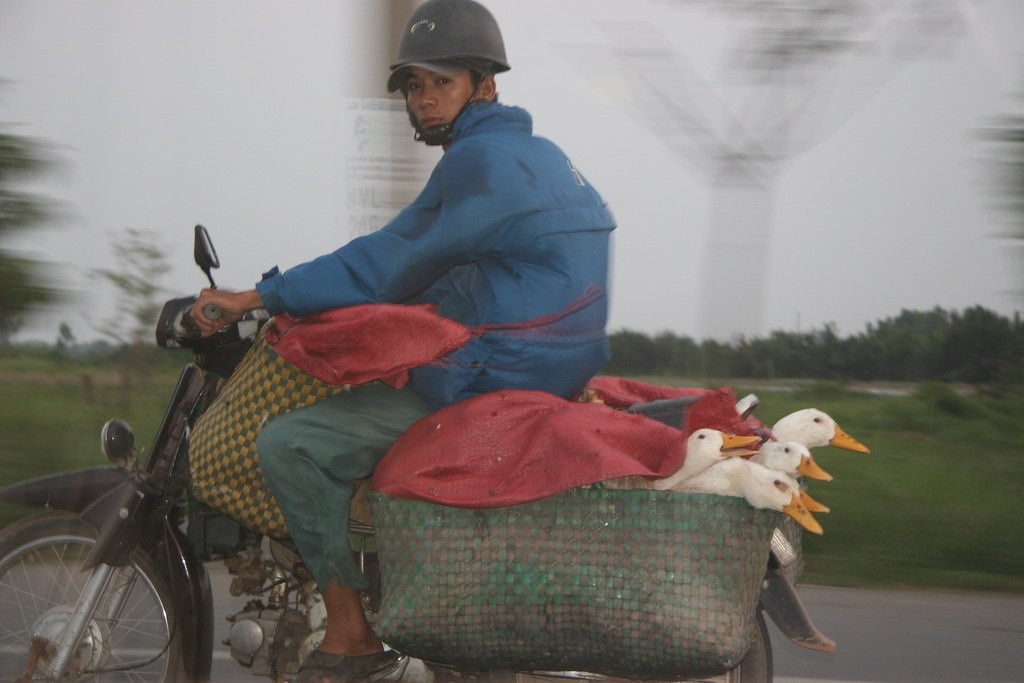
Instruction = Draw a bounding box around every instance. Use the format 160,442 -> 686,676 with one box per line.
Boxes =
190,316 -> 348,539
367,487 -> 776,679
766,476 -> 808,588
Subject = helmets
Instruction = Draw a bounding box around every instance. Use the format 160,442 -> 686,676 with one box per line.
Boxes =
390,0 -> 511,72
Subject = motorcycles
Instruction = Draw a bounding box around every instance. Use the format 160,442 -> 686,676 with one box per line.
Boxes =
0,225 -> 837,683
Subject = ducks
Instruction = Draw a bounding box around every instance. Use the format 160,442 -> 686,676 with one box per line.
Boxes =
600,407 -> 872,537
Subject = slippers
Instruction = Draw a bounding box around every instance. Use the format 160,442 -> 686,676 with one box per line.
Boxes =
293,649 -> 399,683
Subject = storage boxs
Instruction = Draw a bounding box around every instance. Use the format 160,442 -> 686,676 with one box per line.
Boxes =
770,475 -> 804,586
362,485 -> 777,674
186,314 -> 359,539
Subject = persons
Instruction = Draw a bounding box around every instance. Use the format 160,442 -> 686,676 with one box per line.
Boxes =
188,1 -> 612,682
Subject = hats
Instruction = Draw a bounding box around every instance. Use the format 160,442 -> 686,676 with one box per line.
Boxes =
386,62 -> 494,94
